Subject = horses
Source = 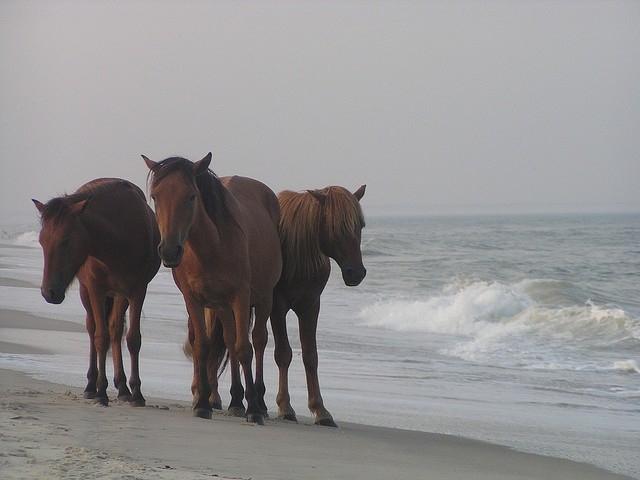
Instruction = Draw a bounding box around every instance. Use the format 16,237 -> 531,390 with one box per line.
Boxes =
207,182 -> 369,429
136,149 -> 285,427
30,177 -> 163,410
